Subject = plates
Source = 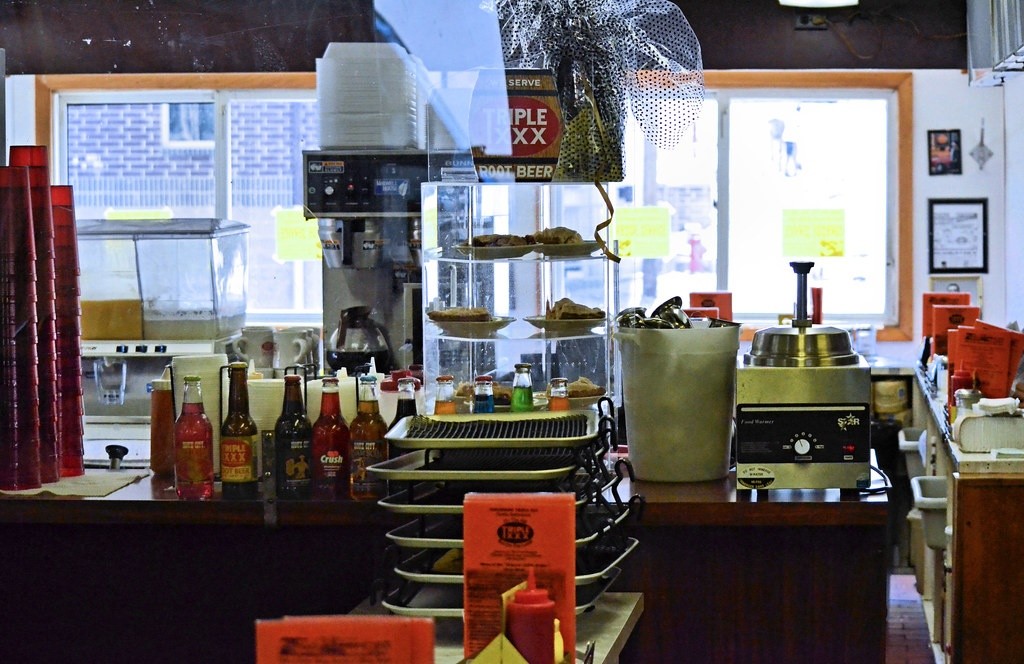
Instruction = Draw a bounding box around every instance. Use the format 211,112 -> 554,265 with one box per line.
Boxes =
455,391 -> 609,413
428,241 -> 607,334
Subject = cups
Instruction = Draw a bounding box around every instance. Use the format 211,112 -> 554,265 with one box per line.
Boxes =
9,145 -> 60,483
233,326 -> 321,373
50,185 -> 85,478
0,165 -> 42,491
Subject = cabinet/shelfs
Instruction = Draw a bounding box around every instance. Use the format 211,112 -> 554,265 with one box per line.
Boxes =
874,372 -> 1024,662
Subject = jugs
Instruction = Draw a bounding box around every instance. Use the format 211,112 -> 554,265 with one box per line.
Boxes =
326,305 -> 392,376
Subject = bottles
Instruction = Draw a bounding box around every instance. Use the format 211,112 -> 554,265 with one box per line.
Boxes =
275,375 -> 313,499
247,359 -> 263,380
223,362 -> 260,501
350,377 -> 388,501
433,375 -> 457,414
313,377 -> 350,499
176,376 -> 214,499
511,364 -> 534,412
549,378 -> 571,409
948,361 -> 981,427
150,380 -> 176,475
388,379 -> 419,435
506,568 -> 556,664
472,375 -> 494,414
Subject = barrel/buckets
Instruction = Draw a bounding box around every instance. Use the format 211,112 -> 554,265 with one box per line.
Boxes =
613,325 -> 743,482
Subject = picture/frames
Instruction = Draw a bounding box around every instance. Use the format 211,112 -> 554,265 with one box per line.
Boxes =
929,197 -> 988,273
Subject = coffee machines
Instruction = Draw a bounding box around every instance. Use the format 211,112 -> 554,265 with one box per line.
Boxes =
302,150 -> 495,376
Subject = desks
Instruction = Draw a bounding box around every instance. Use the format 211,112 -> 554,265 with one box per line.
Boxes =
345,592 -> 646,664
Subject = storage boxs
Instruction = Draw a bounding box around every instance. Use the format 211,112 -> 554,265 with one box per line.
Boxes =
911,474 -> 945,550
900,427 -> 926,483
